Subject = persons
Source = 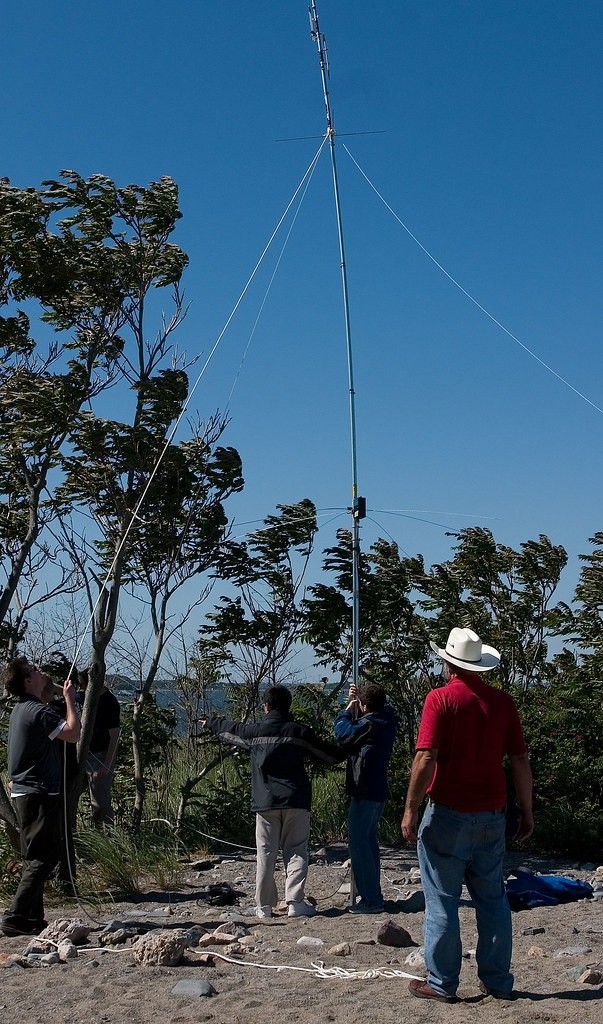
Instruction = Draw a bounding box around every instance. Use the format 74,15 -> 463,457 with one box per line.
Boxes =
401,627 -> 534,1004
76,668 -> 121,838
198,686 -> 348,919
0,656 -> 81,935
333,684 -> 398,914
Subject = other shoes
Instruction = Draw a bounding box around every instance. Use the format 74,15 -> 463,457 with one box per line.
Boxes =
408,979 -> 458,1004
479,980 -> 515,1000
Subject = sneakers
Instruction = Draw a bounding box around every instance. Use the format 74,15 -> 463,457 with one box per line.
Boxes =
287,899 -> 317,917
348,900 -> 385,914
0,911 -> 43,937
253,904 -> 272,918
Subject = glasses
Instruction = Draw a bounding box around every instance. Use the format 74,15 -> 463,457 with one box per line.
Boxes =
27,665 -> 38,675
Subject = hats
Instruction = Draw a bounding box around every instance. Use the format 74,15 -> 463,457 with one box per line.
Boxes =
428,627 -> 501,672
